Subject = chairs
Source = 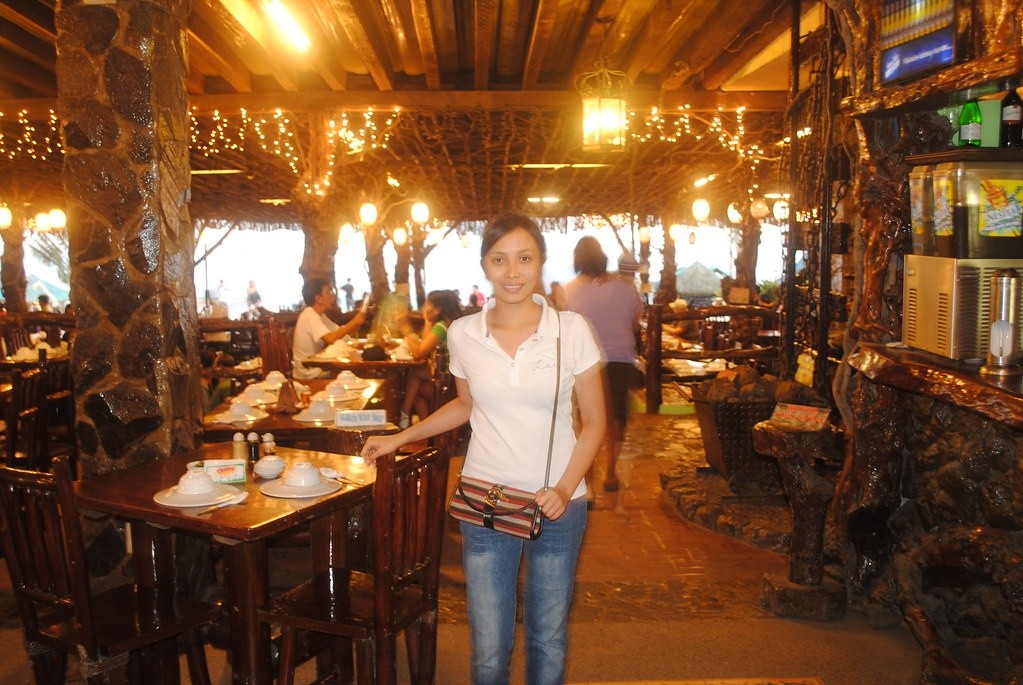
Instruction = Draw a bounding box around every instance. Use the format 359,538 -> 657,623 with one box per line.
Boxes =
0,307 -> 462,685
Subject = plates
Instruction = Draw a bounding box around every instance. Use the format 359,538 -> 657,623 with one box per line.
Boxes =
215,334 -> 422,421
259,477 -> 343,498
185,458 -> 245,482
152,482 -> 240,507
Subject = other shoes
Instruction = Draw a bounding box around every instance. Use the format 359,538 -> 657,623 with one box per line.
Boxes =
603,475 -> 618,491
586,499 -> 595,511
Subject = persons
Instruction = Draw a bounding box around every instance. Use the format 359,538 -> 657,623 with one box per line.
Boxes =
564,235 -> 641,508
455,285 -> 484,315
661,299 -> 700,342
395,290 -> 460,430
360,214 -> 607,685
291,278 -> 364,380
247,282 -> 261,311
545,282 -> 561,304
39,295 -> 53,313
341,278 -> 354,311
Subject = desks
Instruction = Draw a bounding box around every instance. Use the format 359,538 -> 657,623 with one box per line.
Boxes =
0,346 -> 68,372
66,442 -> 409,685
198,376 -> 390,452
302,337 -> 429,424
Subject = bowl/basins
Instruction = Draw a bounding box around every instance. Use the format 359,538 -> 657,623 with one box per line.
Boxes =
177,467 -> 215,494
13,341 -> 57,359
285,462 -> 320,486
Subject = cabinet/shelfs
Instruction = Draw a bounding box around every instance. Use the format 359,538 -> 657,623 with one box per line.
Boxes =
844,52 -> 1022,427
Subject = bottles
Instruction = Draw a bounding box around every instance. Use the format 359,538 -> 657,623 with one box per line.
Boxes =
232,432 -> 245,460
247,432 -> 260,463
958,100 -> 981,147
263,434 -> 276,457
998,88 -> 1023,148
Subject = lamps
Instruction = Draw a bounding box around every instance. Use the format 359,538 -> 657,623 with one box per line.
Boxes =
571,18 -> 631,149
689,225 -> 695,246
0,203 -> 12,230
728,193 -> 789,224
639,225 -> 651,244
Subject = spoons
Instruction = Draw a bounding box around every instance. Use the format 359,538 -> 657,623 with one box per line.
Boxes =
319,467 -> 363,485
196,492 -> 248,517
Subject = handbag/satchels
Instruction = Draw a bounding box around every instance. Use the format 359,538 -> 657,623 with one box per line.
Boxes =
449,475 -> 544,540
